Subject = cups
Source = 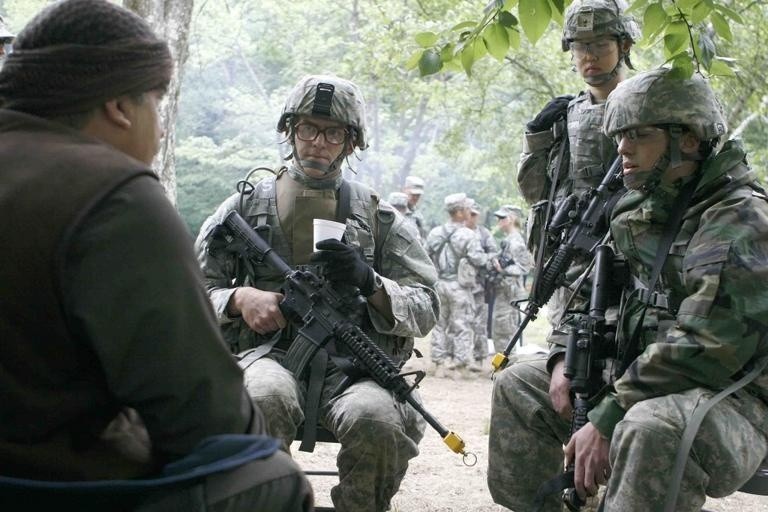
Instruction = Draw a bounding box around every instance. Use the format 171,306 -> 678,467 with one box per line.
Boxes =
311,218 -> 345,254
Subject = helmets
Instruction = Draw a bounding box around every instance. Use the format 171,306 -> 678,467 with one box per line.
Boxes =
276,74 -> 369,151
602,66 -> 727,140
560,0 -> 641,53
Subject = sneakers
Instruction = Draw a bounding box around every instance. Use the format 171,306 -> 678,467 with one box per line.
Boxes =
435,364 -> 479,380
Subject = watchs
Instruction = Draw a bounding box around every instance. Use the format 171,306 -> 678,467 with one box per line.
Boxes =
365,268 -> 384,298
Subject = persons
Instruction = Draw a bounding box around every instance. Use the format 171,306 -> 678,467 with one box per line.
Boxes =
0,2 -> 313,510
484,64 -> 767,511
515,0 -> 639,335
190,73 -> 440,511
384,177 -> 536,379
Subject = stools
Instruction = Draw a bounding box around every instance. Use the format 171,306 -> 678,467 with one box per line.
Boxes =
288,423 -> 345,511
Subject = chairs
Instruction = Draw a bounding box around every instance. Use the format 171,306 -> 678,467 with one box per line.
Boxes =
0,428 -> 283,509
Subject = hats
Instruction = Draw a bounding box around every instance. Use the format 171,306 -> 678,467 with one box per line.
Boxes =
389,192 -> 409,206
405,176 -> 425,195
493,204 -> 522,219
470,203 -> 481,214
444,193 -> 474,209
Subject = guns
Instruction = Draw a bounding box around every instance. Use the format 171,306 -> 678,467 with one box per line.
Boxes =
484,258 -> 513,339
214,209 -> 476,467
487,148 -> 626,371
559,244 -> 625,509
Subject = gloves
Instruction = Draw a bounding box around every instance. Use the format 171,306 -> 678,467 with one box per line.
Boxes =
310,238 -> 375,297
527,94 -> 576,134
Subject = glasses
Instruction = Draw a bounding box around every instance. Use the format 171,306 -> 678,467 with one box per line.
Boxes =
612,126 -> 691,144
293,120 -> 350,145
568,38 -> 617,61
